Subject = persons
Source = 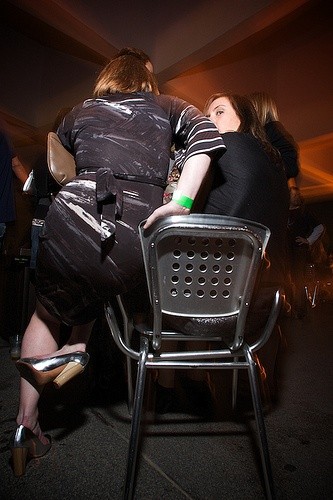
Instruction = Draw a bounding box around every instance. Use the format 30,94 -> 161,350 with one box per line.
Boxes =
0,129 -> 33,244
146,92 -> 323,411
11,49 -> 226,478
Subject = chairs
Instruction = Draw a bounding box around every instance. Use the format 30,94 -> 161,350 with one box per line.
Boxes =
105,212 -> 287,500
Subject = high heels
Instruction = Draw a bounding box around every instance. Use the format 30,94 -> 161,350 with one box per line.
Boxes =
9,424 -> 52,476
15,351 -> 89,388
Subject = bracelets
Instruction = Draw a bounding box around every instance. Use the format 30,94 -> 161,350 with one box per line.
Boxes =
170,192 -> 195,208
289,186 -> 299,192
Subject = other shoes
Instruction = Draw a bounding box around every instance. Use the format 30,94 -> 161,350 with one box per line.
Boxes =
293,314 -> 305,321
154,380 -> 176,415
10,334 -> 22,358
180,376 -> 215,417
